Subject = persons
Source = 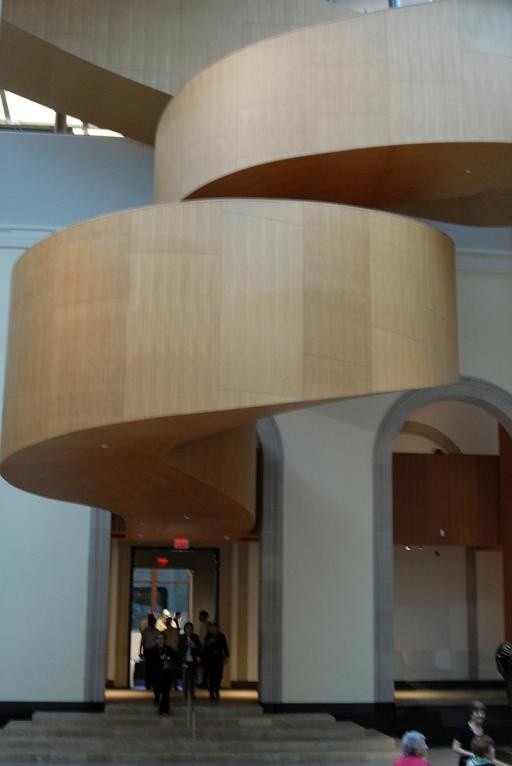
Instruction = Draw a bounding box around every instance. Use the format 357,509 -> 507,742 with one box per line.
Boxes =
453,701 -> 506,766
393,730 -> 430,766
139,609 -> 229,718
